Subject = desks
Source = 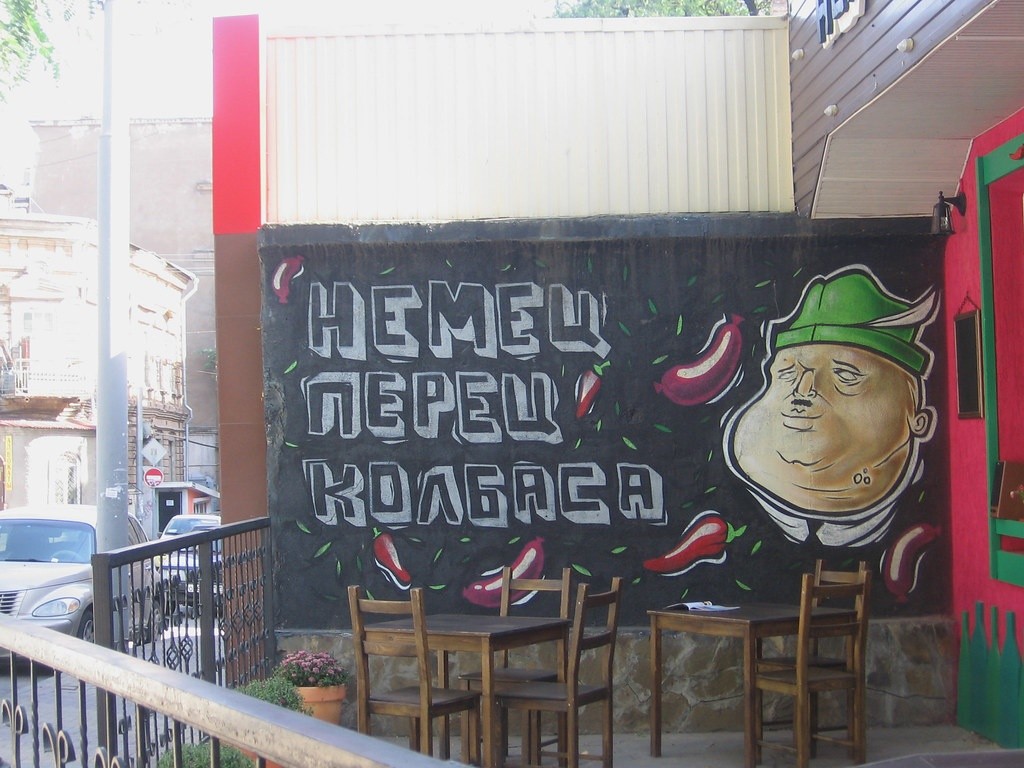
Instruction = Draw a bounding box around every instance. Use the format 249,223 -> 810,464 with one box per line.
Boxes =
363,612 -> 571,768
646,604 -> 858,768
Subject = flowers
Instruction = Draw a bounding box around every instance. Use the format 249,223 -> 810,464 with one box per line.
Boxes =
281,649 -> 348,688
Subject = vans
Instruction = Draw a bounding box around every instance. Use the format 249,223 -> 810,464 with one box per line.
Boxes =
0,503 -> 161,658
156,514 -> 223,617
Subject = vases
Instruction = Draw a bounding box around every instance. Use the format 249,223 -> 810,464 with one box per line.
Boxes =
296,685 -> 346,724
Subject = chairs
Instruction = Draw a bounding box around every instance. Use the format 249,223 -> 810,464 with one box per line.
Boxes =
492,577 -> 622,768
347,585 -> 481,766
748,571 -> 869,768
755,559 -> 868,757
457,564 -> 571,768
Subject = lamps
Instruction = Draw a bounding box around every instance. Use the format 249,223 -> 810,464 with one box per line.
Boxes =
925,190 -> 968,233
